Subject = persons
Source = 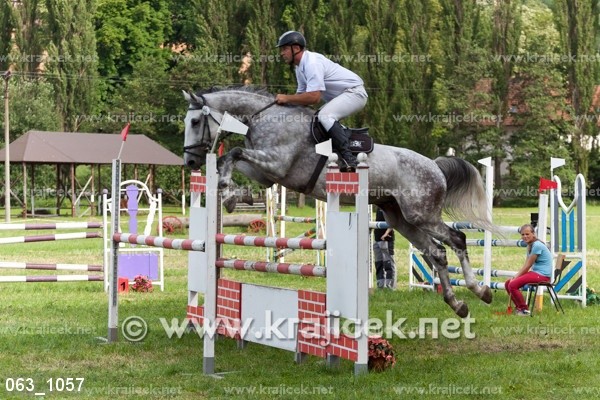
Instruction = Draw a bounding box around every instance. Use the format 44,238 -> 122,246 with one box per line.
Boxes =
504,222 -> 554,314
372,208 -> 398,293
274,29 -> 369,173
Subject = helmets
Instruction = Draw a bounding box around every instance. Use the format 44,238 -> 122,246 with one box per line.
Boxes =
275,31 -> 305,72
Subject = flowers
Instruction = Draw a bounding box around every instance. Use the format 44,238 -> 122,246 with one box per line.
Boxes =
368,337 -> 396,369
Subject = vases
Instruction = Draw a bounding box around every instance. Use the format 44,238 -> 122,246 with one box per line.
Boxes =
370,356 -> 389,371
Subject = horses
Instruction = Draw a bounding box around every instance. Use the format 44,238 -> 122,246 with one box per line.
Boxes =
181,84 -> 509,318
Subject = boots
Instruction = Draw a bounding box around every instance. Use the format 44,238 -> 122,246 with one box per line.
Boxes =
327,122 -> 359,171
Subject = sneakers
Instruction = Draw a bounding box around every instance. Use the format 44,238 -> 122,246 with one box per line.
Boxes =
517,309 -> 531,317
